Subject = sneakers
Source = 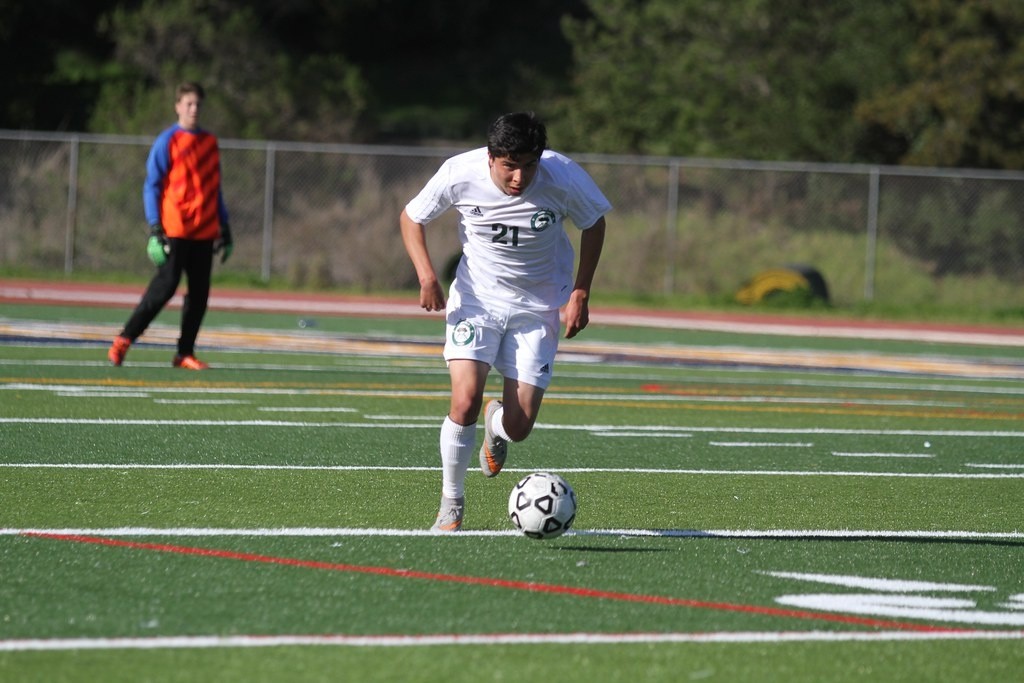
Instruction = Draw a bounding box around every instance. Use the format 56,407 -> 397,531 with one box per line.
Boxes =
108,336 -> 130,367
172,354 -> 208,370
479,400 -> 508,477
431,503 -> 464,532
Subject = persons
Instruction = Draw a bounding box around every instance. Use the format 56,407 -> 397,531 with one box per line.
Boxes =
108,81 -> 232,371
400,112 -> 612,531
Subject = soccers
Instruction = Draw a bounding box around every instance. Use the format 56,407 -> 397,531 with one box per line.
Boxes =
506,471 -> 579,541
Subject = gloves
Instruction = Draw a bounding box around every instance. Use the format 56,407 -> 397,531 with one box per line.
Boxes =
213,224 -> 233,265
146,225 -> 171,266
